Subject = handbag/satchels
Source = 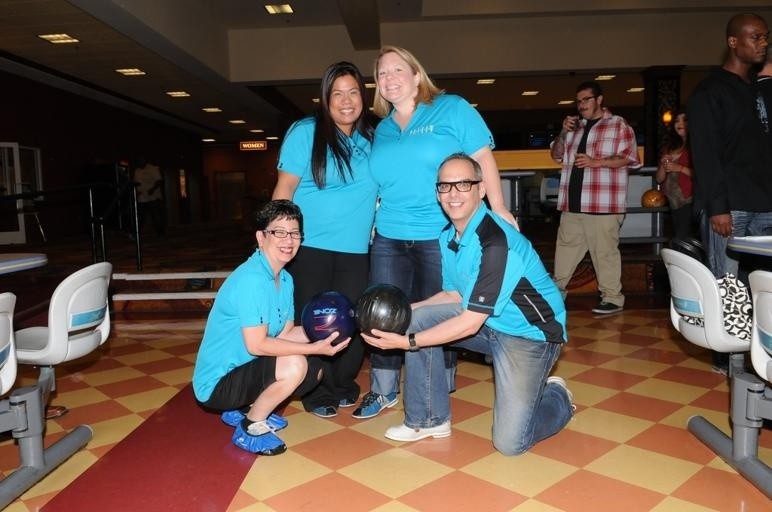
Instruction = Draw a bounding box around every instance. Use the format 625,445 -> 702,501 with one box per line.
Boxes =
684,272 -> 753,341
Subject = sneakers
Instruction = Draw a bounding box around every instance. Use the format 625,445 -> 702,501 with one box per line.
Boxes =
385,420 -> 452,442
222,406 -> 287,455
592,300 -> 623,314
547,376 -> 576,414
311,391 -> 400,419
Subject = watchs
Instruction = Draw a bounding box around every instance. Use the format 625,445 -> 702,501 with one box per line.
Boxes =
408,333 -> 419,353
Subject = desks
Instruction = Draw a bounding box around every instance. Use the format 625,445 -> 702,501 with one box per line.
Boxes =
729,235 -> 772,259
1,251 -> 48,274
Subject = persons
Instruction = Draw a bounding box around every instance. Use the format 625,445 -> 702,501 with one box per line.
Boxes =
359,151 -> 578,458
270,60 -> 380,418
130,152 -> 166,245
550,79 -> 642,315
687,9 -> 772,377
655,104 -> 698,238
349,41 -> 521,419
192,199 -> 353,456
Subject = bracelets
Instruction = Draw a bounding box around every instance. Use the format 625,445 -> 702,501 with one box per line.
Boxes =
679,165 -> 685,173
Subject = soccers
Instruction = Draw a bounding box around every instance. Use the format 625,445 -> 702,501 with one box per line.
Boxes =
356,282 -> 412,334
302,291 -> 355,346
643,192 -> 666,210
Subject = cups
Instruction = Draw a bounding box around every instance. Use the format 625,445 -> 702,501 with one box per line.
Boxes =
570,116 -> 579,129
663,154 -> 672,164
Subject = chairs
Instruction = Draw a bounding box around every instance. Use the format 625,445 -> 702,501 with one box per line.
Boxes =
662,247 -> 750,353
748,271 -> 772,384
0,292 -> 18,396
14,262 -> 112,365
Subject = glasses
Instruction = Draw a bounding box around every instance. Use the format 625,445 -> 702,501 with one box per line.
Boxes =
574,96 -> 594,105
263,229 -> 303,239
435,181 -> 481,194
